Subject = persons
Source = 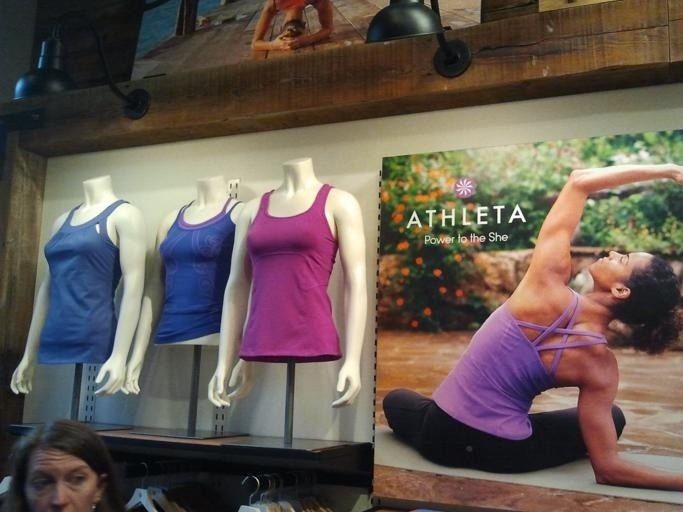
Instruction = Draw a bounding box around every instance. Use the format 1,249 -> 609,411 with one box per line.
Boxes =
8,419 -> 126,512
383,164 -> 683,491
252,0 -> 334,50
12,175 -> 146,397
209,159 -> 365,406
121,178 -> 254,400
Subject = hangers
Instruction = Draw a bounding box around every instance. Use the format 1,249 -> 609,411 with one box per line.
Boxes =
117,461 -> 189,511
236,470 -> 303,511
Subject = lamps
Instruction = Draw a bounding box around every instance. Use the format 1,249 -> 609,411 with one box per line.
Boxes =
366,0 -> 472,79
12,10 -> 151,122
